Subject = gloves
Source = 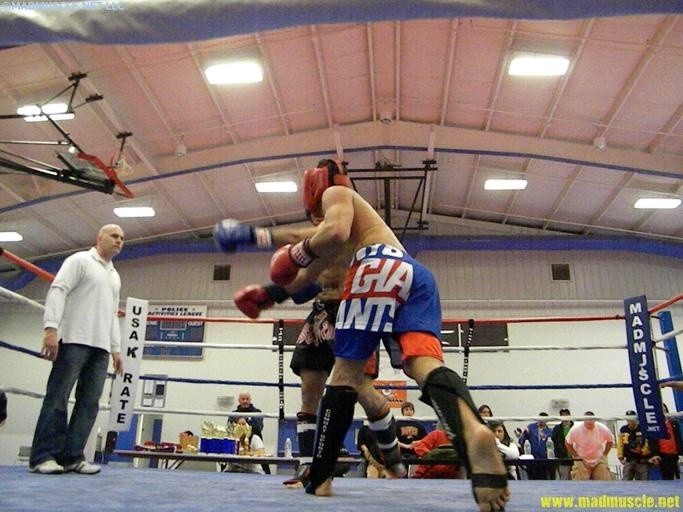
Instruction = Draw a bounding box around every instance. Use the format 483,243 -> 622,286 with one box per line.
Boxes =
232,283 -> 290,319
269,238 -> 319,286
211,219 -> 273,255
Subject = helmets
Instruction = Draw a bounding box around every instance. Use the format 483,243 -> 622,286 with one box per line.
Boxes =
303,158 -> 354,226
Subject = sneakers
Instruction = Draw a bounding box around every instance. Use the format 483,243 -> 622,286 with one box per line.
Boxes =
29,459 -> 64,475
64,458 -> 102,475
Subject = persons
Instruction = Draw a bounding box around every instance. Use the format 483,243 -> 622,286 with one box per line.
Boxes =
231,157 -> 511,510
28,222 -> 129,476
221,415 -> 265,475
226,390 -> 271,475
354,401 -> 683,481
211,203 -> 406,485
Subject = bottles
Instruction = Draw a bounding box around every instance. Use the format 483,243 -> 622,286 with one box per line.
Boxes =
523,439 -> 530,455
546,436 -> 555,459
284,437 -> 292,457
